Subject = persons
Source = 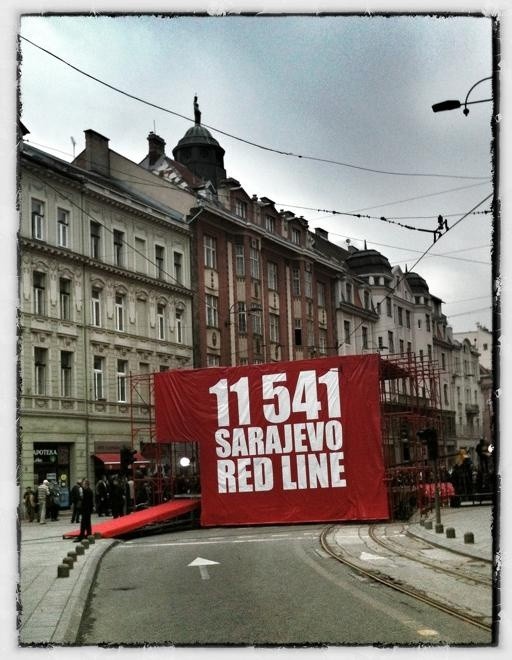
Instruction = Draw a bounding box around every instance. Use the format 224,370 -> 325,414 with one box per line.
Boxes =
386,437 -> 493,503
22,463 -> 202,543
